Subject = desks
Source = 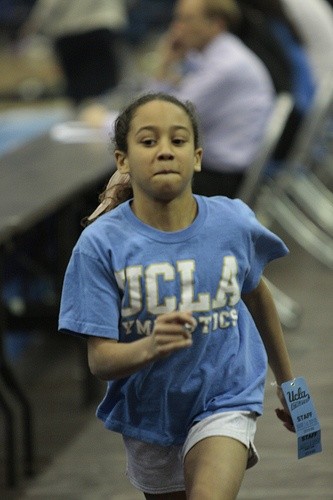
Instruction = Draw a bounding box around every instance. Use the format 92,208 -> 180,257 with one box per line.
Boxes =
0,82 -> 146,497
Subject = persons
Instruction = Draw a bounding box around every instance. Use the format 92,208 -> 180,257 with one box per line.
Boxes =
29,0 -> 128,118
143,0 -> 278,200
56,90 -> 300,500
233,0 -> 315,181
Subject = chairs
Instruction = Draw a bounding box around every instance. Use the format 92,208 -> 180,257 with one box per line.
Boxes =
229,88 -> 333,332
54,28 -> 123,106
275,78 -> 333,235
